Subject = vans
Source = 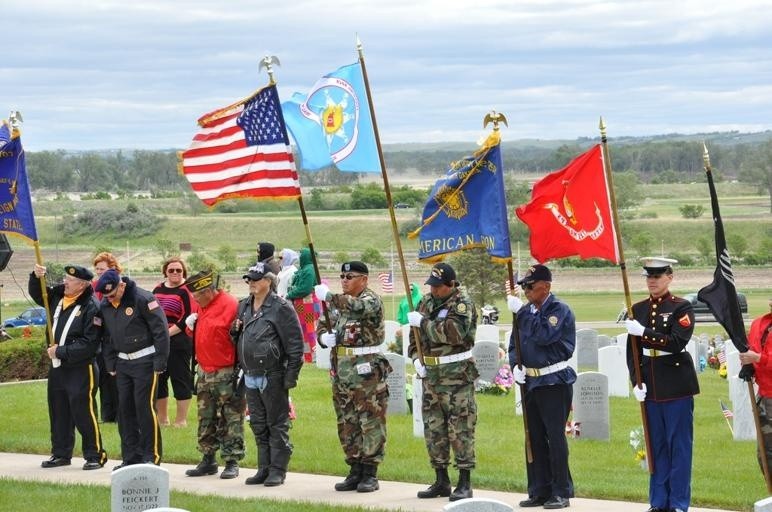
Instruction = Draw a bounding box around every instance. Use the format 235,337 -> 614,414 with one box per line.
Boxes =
680,293 -> 750,320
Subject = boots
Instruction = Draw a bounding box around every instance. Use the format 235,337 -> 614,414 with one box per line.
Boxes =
186,455 -> 218,476
264,449 -> 290,486
450,469 -> 472,501
418,468 -> 451,498
357,465 -> 379,492
335,465 -> 362,491
246,445 -> 270,484
220,460 -> 239,479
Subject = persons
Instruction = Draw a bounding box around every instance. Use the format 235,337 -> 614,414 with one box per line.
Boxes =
405,263 -> 478,501
255,242 -> 322,362
624,254 -> 701,511
315,261 -> 393,493
27,251 -> 305,487
505,263 -> 577,508
396,282 -> 423,326
0,325 -> 32,343
735,301 -> 772,495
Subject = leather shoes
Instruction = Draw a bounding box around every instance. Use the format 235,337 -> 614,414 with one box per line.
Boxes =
82,462 -> 101,470
544,496 -> 569,509
42,456 -> 71,467
520,495 -> 549,507
648,505 -> 669,511
671,508 -> 684,512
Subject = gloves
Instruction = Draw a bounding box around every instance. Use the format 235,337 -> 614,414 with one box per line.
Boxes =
185,313 -> 198,331
284,380 -> 296,390
513,364 -> 526,384
508,295 -> 524,313
633,383 -> 647,401
314,284 -> 328,302
231,320 -> 240,335
414,359 -> 427,377
625,319 -> 645,337
321,329 -> 337,347
408,311 -> 423,327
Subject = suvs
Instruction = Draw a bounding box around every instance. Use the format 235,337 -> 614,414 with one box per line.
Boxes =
2,308 -> 46,328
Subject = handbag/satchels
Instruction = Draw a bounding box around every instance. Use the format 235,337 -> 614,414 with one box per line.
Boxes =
231,363 -> 244,393
191,360 -> 198,395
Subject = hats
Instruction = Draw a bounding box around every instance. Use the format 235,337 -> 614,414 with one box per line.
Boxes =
640,258 -> 677,276
341,261 -> 368,274
424,263 -> 456,287
517,264 -> 552,284
184,270 -> 213,292
64,266 -> 93,281
243,262 -> 272,280
95,269 -> 120,294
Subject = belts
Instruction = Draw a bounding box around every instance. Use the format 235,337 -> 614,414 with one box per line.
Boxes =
50,299 -> 81,368
423,350 -> 472,365
526,361 -> 567,376
118,345 -> 156,360
336,346 -> 379,355
643,348 -> 685,357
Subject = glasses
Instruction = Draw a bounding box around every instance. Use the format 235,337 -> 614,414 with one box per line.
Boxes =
104,288 -> 118,298
168,269 -> 182,273
248,278 -> 259,281
191,294 -> 204,303
522,283 -> 532,289
340,273 -> 363,280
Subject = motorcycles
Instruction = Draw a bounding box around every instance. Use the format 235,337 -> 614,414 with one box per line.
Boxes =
616,302 -> 629,324
481,305 -> 499,324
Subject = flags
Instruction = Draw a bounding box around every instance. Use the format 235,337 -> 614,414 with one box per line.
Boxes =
277,61 -> 383,176
1,129 -> 40,244
416,132 -> 514,266
503,269 -> 520,297
515,144 -> 621,268
719,399 -> 733,418
172,82 -> 302,210
0,123 -> 12,150
714,351 -> 727,366
697,168 -> 755,380
